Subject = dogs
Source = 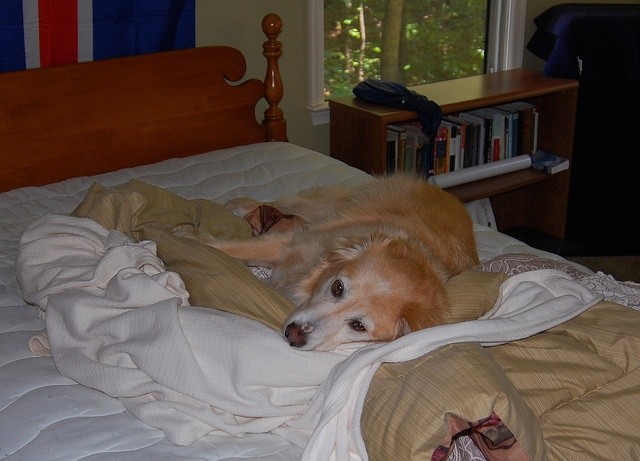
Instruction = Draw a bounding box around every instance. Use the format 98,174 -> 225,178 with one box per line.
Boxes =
165,165 -> 482,353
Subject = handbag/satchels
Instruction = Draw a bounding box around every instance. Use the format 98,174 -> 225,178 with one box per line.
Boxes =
353,79 -> 442,138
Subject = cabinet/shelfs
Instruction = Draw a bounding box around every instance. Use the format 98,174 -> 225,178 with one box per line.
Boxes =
324,67 -> 578,241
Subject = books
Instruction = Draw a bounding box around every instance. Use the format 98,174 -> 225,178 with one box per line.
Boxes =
464,197 -> 498,231
385,100 -> 570,180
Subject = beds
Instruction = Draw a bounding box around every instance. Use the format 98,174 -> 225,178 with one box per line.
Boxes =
0,12 -> 640,460
